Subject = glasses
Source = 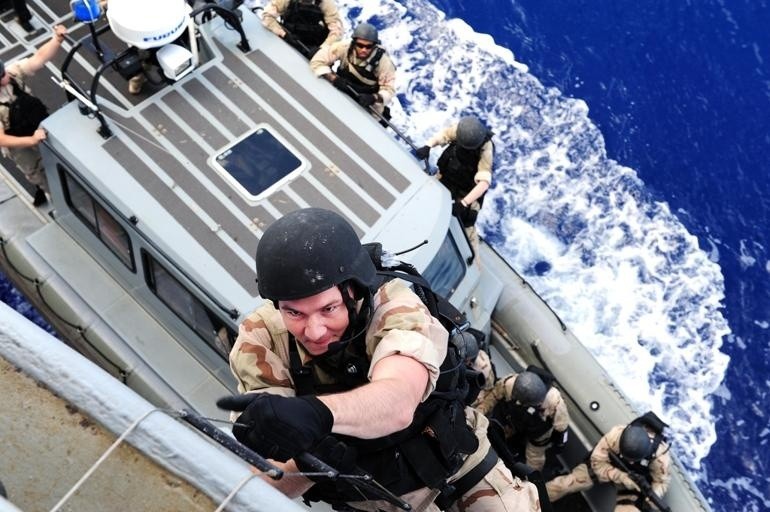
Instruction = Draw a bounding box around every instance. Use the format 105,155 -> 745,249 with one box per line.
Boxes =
355,42 -> 374,49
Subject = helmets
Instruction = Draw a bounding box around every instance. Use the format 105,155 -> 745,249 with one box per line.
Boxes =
256,208 -> 377,302
352,24 -> 378,43
620,427 -> 651,458
451,332 -> 478,362
511,372 -> 546,406
456,117 -> 487,150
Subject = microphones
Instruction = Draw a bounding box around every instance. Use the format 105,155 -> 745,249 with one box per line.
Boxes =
327,291 -> 374,354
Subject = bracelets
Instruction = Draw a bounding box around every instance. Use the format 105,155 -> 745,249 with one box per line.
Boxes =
462,199 -> 470,206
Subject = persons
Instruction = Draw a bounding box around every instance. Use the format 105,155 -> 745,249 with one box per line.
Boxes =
542,412 -> 672,511
214,208 -> 541,512
309,23 -> 397,129
0,24 -> 69,208
414,116 -> 495,276
259,0 -> 345,59
473,366 -> 569,475
450,332 -> 495,407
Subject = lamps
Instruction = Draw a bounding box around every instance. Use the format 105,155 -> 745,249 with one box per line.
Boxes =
156,43 -> 195,82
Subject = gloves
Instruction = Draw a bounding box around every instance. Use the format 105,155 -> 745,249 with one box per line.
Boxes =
217,393 -> 335,464
620,473 -> 641,492
415,145 -> 430,159
358,94 -> 376,106
452,202 -> 468,216
282,33 -> 301,46
333,77 -> 354,93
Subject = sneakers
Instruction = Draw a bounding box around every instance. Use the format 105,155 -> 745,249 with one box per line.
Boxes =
34,185 -> 46,207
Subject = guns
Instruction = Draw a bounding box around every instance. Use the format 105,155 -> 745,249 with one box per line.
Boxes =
608,449 -> 671,512
